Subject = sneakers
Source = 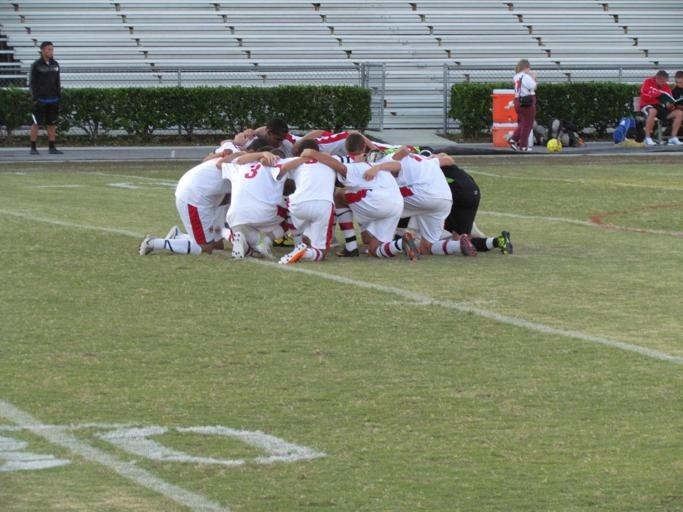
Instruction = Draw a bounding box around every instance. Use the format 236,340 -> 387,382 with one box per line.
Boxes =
30,149 -> 39,154
459,233 -> 478,257
48,147 -> 64,154
277,243 -> 308,265
138,234 -> 154,256
231,231 -> 250,259
520,147 -> 532,151
644,137 -> 657,146
164,226 -> 180,239
335,247 -> 359,257
507,137 -> 518,151
496,231 -> 512,255
401,232 -> 419,261
666,136 -> 683,145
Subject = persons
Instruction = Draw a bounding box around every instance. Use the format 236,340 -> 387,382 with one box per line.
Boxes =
138,116 -> 514,265
640,70 -> 683,146
666,70 -> 683,110
506,58 -> 538,152
28,40 -> 64,155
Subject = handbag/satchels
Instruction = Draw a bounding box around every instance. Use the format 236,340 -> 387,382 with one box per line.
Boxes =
519,95 -> 532,106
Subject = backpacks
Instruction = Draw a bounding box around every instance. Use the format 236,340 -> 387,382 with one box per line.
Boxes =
614,117 -> 636,144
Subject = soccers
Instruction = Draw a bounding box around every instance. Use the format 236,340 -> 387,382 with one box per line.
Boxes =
547,139 -> 561,152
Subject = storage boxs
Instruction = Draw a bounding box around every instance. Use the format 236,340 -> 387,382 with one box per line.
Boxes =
492,122 -> 534,146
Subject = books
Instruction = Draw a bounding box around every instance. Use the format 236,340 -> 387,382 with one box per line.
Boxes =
659,89 -> 683,105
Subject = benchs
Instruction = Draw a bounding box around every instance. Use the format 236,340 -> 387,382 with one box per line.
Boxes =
0,0 -> 683,129
